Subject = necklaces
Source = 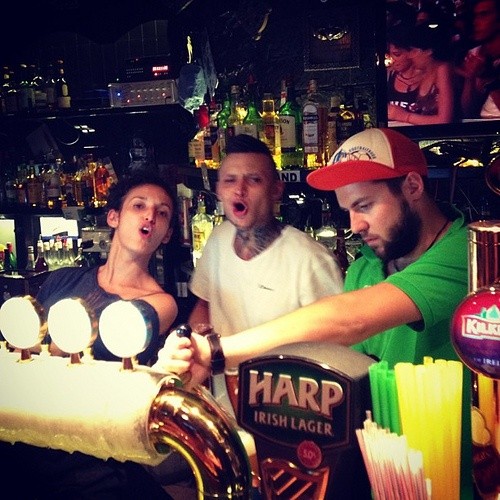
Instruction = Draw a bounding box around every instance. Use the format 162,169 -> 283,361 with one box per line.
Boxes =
396,72 -> 425,93
392,218 -> 450,273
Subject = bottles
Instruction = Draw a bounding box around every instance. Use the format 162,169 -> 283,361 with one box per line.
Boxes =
0,237 -> 97,305
167,78 -> 377,287
0,153 -> 115,220
0,60 -> 73,113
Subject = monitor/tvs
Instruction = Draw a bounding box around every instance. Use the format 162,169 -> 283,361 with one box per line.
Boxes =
374,0 -> 500,139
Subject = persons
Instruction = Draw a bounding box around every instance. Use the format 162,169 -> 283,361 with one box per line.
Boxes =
33,173 -> 179,367
186,133 -> 344,337
148,128 -> 468,393
383,0 -> 500,128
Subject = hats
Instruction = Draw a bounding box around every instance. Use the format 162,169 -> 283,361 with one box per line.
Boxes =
304,127 -> 429,192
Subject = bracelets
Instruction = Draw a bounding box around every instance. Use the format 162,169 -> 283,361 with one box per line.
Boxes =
406,112 -> 412,123
192,321 -> 226,375
